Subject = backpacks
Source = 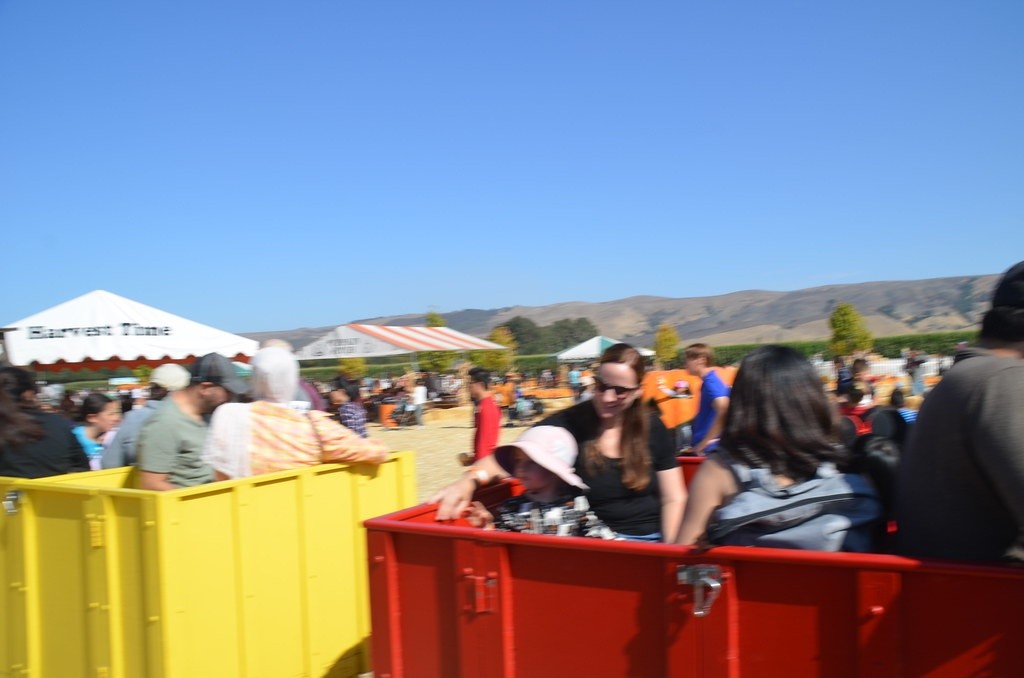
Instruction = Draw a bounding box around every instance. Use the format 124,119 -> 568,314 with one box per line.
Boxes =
706,444 -> 888,552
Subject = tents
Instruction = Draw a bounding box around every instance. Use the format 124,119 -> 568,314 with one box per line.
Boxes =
293,323 -> 507,361
557,336 -> 657,364
0,290 -> 260,373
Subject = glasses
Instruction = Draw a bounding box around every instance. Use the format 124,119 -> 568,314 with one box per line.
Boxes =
592,376 -> 639,396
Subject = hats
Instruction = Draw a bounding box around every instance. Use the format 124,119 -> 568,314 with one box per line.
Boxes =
901,357 -> 924,368
189,352 -> 249,394
674,381 -> 689,387
148,362 -> 191,392
578,376 -> 596,387
492,425 -> 589,491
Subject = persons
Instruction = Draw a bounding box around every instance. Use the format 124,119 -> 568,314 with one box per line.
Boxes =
891,261 -> 1024,567
428,341 -> 969,554
0,349 -> 427,490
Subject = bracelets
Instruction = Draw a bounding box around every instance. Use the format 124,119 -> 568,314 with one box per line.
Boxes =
465,468 -> 480,490
